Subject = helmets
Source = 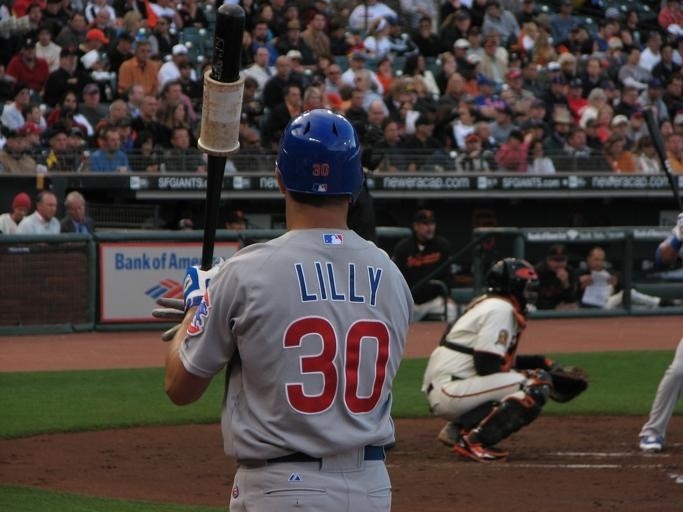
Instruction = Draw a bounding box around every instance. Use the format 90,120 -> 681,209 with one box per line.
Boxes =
274,107 -> 365,198
490,255 -> 540,301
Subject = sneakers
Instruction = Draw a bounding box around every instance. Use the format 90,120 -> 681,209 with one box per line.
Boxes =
453,430 -> 514,464
639,433 -> 665,452
437,420 -> 460,444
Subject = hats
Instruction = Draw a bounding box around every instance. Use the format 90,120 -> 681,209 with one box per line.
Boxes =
451,9 -> 483,48
479,37 -> 663,129
547,244 -> 568,260
286,49 -> 370,63
413,210 -> 437,224
20,25 -> 134,131
11,192 -> 33,210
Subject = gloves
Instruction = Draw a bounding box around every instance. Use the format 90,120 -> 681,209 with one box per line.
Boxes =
180,262 -> 225,308
152,299 -> 186,343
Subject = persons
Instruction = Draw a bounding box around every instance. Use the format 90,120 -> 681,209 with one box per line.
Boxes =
153,108 -> 417,510
0,3 -> 682,318
636,211 -> 683,453
419,256 -> 591,467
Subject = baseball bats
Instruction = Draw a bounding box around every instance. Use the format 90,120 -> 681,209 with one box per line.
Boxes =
641,107 -> 683,212
198,5 -> 246,271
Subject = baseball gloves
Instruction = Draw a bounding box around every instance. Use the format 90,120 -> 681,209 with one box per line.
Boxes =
548,363 -> 588,402
